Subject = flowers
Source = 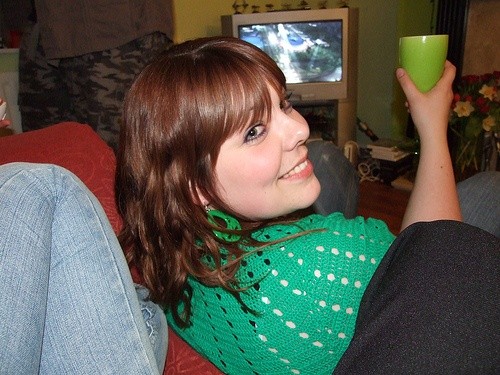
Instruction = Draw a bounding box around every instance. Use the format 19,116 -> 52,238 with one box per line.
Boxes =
449,69 -> 500,175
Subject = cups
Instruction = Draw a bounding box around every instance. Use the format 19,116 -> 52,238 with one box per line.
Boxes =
399,35 -> 449,94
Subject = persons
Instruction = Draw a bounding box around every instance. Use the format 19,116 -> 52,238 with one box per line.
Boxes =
329,218 -> 500,375
1,161 -> 169,375
0,0 -> 176,158
119,32 -> 471,375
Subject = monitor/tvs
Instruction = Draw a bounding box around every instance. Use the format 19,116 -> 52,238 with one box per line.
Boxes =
221,7 -> 357,101
